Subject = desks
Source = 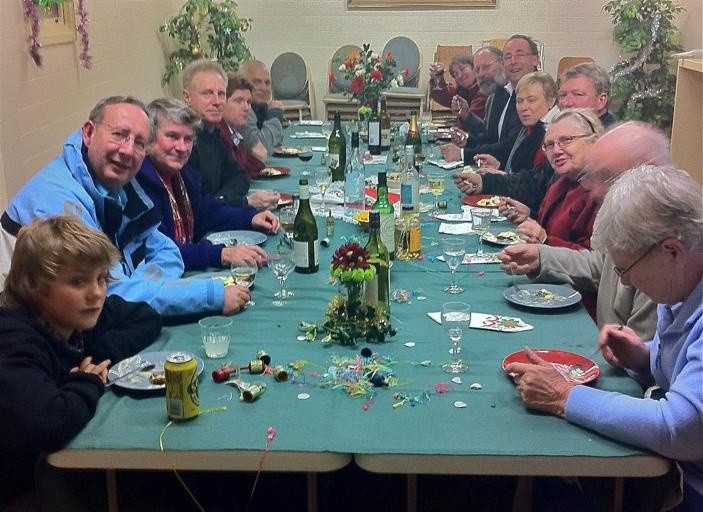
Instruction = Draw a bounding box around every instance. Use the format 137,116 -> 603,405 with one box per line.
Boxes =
668,57 -> 703,184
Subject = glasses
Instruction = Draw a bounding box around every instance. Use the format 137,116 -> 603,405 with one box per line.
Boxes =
95,120 -> 152,155
502,51 -> 537,62
541,132 -> 592,151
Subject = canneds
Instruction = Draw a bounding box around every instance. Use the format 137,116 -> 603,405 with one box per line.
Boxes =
164,353 -> 200,421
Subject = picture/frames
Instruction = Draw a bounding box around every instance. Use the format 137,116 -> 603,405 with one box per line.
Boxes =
25,1 -> 77,47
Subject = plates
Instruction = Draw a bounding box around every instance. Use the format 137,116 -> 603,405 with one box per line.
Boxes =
501,348 -> 600,385
206,229 -> 267,248
259,165 -> 291,178
462,193 -> 508,207
108,352 -> 204,393
428,118 -> 458,142
186,271 -> 254,292
270,146 -> 301,156
273,191 -> 293,207
481,225 -> 523,246
502,284 -> 582,309
289,130 -> 325,139
295,120 -> 323,126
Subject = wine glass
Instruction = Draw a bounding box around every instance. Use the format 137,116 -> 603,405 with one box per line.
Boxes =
439,237 -> 465,294
277,207 -> 298,256
230,255 -> 258,308
265,246 -> 296,307
297,142 -> 313,176
426,172 -> 445,215
420,109 -> 438,163
440,302 -> 471,374
470,207 -> 493,260
430,62 -> 444,90
312,165 -> 332,215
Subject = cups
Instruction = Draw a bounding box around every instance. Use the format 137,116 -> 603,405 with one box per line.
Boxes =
198,316 -> 233,359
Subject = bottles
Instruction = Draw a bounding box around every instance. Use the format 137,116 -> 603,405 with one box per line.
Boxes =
328,96 -> 422,216
369,170 -> 395,261
291,178 -> 319,273
326,208 -> 334,236
359,209 -> 391,323
396,204 -> 421,260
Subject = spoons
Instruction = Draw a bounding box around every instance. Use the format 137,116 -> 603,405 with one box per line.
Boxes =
509,256 -> 521,293
457,98 -> 462,115
462,178 -> 479,189
104,360 -> 156,387
563,324 -> 624,375
229,238 -> 239,246
506,201 -> 516,216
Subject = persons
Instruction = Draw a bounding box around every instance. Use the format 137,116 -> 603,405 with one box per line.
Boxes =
0,214 -> 160,511
503,163 -> 703,511
425,35 -> 667,346
0,56 -> 288,318
